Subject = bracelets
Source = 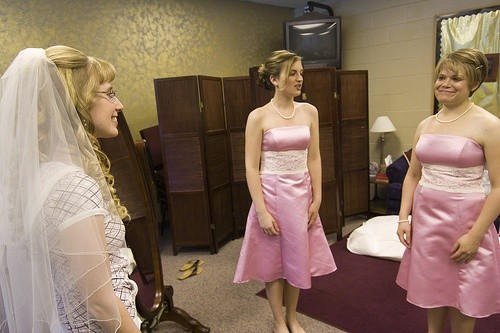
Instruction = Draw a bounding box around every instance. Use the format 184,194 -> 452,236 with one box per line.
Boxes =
397,220 -> 410,223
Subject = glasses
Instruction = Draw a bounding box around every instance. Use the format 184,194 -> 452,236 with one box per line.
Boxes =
96,87 -> 117,102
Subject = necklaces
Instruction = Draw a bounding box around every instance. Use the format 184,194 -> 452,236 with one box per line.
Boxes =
270,98 -> 296,119
436,101 -> 474,123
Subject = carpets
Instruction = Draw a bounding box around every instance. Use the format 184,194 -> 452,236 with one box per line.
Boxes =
256,221 -> 500,333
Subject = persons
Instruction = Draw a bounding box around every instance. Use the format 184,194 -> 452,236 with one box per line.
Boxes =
234,50 -> 337,333
397,49 -> 500,333
0,45 -> 143,333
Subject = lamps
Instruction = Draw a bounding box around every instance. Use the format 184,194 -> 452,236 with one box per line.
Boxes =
369,116 -> 397,171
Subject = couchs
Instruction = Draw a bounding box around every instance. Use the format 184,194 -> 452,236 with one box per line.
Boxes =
386,149 -> 413,215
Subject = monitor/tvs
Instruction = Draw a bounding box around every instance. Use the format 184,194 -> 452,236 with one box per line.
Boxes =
284,15 -> 341,69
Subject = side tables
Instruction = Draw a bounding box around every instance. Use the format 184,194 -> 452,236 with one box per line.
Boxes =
370,174 -> 389,216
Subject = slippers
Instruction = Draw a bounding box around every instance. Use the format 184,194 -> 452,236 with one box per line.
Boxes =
178,266 -> 203,280
179,260 -> 204,271
